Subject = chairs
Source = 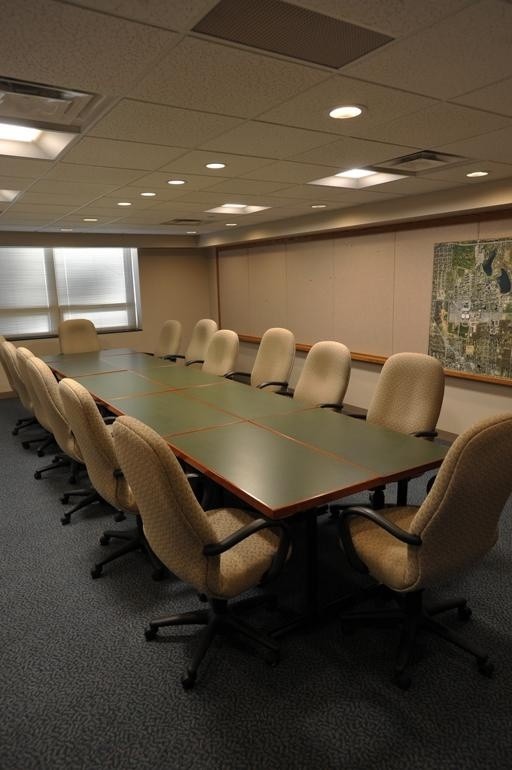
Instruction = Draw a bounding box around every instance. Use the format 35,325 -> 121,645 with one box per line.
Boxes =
164,318 -> 217,368
186,330 -> 240,379
57,320 -> 102,354
2,335 -> 115,490
341,410 -> 512,679
278,339 -> 351,412
367,351 -> 446,442
227,327 -> 296,393
144,320 -> 183,361
106,414 -> 294,690
54,379 -> 189,585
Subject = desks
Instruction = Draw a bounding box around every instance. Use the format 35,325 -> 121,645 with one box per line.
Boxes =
33,348 -> 450,665
